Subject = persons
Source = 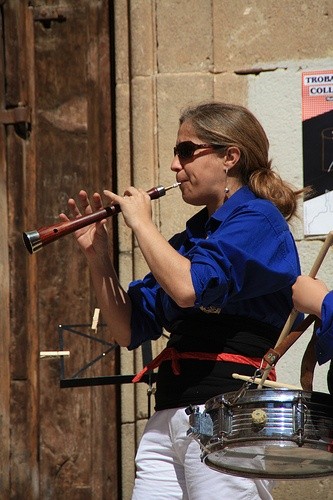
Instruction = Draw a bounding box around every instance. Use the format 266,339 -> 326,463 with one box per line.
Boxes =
292,275 -> 333,395
58,102 -> 305,500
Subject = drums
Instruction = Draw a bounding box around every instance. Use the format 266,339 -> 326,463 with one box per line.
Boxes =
187,384 -> 333,480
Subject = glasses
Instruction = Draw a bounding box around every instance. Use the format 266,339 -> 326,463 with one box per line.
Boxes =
173,141 -> 228,164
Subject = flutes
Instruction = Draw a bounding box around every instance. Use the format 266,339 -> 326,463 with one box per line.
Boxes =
21,182 -> 182,257
